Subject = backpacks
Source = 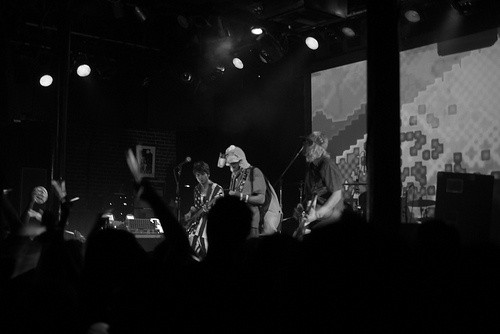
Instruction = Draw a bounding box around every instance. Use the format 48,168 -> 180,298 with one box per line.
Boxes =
248,166 -> 283,236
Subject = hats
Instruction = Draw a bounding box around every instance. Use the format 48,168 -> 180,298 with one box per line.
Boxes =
225,145 -> 250,175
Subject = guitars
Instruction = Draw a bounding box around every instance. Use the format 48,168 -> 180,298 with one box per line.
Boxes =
183,202 -> 213,234
292,194 -> 320,242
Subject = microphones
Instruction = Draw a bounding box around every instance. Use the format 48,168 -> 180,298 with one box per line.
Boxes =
178,156 -> 191,169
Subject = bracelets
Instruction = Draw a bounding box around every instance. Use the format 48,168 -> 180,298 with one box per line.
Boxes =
245,195 -> 249,202
240,193 -> 243,200
190,210 -> 194,215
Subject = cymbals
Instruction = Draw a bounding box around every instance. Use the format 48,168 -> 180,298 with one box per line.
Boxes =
404,199 -> 437,207
342,183 -> 370,185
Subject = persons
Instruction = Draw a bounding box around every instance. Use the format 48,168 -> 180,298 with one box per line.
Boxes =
0,131 -> 500,334
